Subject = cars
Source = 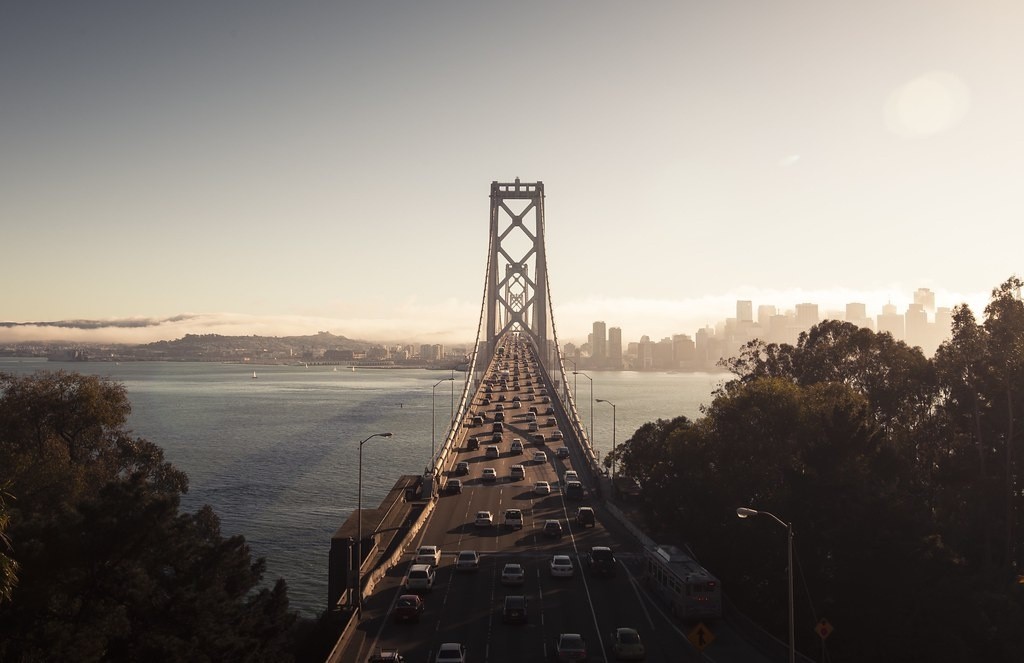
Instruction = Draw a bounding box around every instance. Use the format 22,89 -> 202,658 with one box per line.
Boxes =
533,480 -> 551,495
456,550 -> 481,571
502,595 -> 529,625
481,468 -> 496,482
475,510 -> 493,528
564,480 -> 583,501
415,546 -> 441,567
504,508 -> 523,530
380,648 -> 403,663
455,461 -> 469,476
510,438 -> 523,455
473,332 -> 558,431
584,546 -> 617,576
551,430 -> 564,440
446,479 -> 463,494
575,507 -> 595,528
501,564 -> 525,584
610,627 -> 645,659
553,633 -> 587,663
435,643 -> 466,663
485,445 -> 500,459
556,447 -> 570,459
492,431 -> 503,443
541,518 -> 564,539
509,464 -> 526,480
533,433 -> 546,446
466,437 -> 480,451
563,471 -> 579,483
549,555 -> 574,577
393,594 -> 424,623
532,451 -> 547,463
406,564 -> 436,591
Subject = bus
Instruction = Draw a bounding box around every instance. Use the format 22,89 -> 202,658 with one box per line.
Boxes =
645,544 -> 724,623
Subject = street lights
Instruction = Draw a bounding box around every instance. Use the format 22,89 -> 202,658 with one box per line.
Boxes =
549,333 -> 615,500
346,525 -> 412,609
737,507 -> 794,662
432,356 -> 485,473
357,432 -> 393,620
